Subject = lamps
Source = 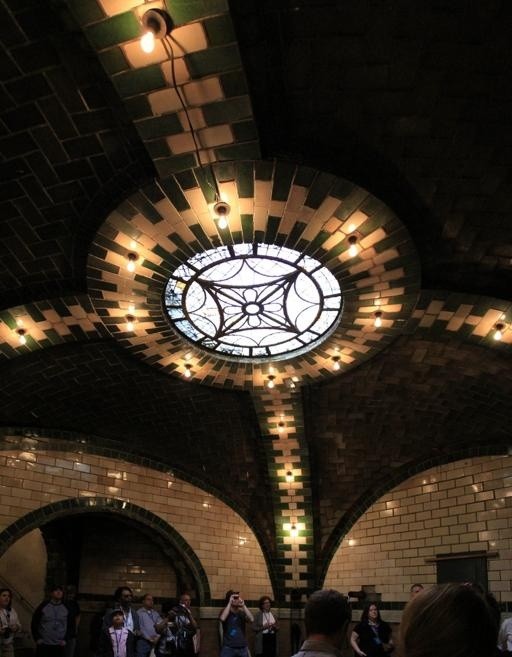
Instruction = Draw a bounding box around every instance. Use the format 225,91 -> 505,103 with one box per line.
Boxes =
277,417 -> 302,541
137,9 -> 169,54
491,322 -> 506,343
212,200 -> 234,232
119,246 -> 202,381
262,372 -> 276,389
16,327 -> 27,346
331,233 -> 385,372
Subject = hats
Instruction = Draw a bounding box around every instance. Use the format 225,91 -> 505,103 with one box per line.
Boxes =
110,608 -> 124,617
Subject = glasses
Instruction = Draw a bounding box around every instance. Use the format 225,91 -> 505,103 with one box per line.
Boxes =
113,612 -> 123,616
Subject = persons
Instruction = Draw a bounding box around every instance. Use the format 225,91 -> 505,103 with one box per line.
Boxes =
219,590 -> 254,657
68,583 -> 81,657
97,586 -> 196,656
31,585 -> 73,656
411,584 -> 426,598
0,588 -> 22,656
498,618 -> 512,654
398,584 -> 499,656
291,589 -> 351,656
351,603 -> 394,657
252,596 -> 279,656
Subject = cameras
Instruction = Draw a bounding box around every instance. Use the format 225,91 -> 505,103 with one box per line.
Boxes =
234,596 -> 239,600
176,604 -> 186,616
3,625 -> 12,639
